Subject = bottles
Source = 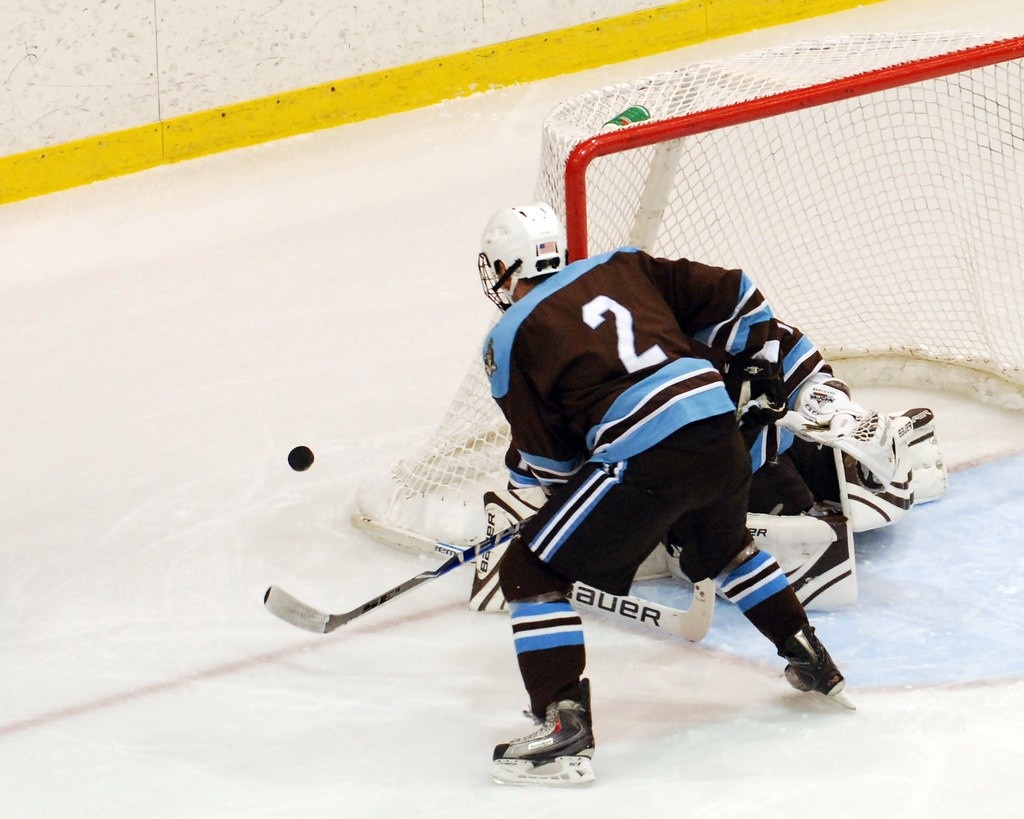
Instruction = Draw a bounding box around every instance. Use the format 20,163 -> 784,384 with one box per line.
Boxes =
601,105 -> 652,135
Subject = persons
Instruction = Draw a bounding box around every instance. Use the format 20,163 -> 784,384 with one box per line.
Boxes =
468,321 -> 948,613
475,198 -> 856,790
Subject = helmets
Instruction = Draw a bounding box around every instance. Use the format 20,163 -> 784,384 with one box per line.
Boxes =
477,201 -> 569,314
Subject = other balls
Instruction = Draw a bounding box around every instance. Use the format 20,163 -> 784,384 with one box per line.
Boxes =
287,443 -> 315,473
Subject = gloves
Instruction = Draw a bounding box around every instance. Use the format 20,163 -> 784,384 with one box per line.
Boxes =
721,337 -> 789,437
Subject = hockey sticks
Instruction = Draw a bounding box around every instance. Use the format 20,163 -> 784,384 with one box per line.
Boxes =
348,509 -> 716,646
261,392 -> 774,637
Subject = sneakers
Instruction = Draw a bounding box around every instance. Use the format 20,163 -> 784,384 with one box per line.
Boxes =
781,624 -> 857,709
490,678 -> 597,790
